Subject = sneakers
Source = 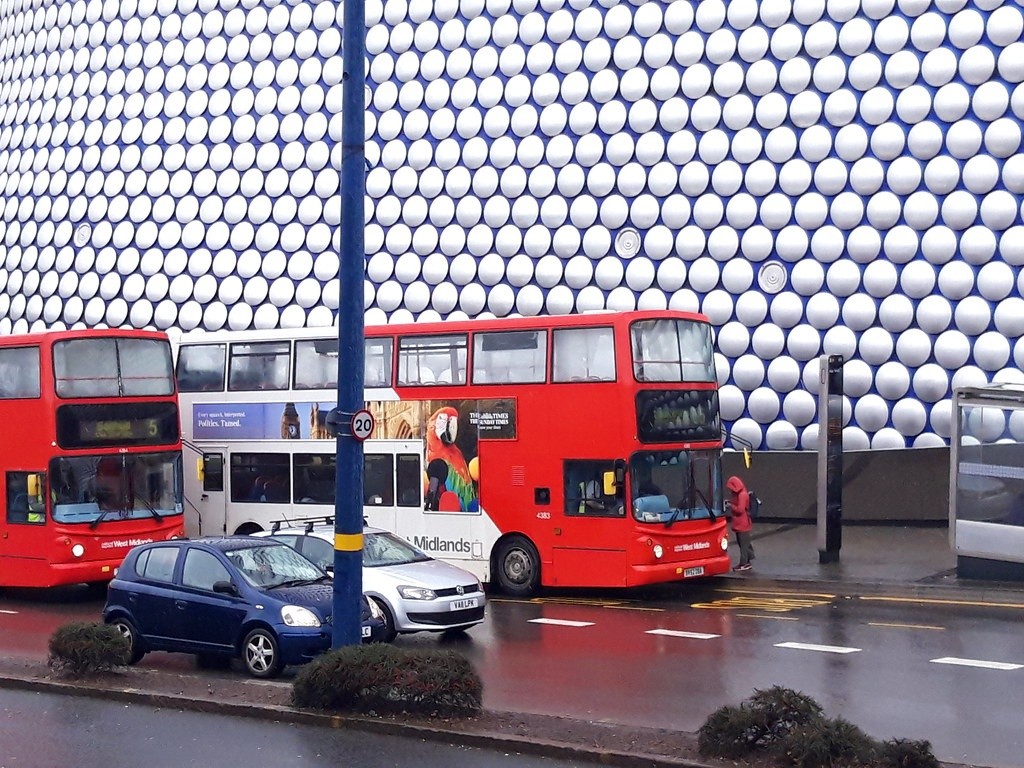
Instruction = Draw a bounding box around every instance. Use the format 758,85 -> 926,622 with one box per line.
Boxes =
734,562 -> 752,571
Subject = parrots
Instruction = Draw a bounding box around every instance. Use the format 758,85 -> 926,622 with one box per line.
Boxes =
423,406 -> 476,512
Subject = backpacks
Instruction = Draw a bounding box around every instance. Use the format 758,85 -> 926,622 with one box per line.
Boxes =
746,491 -> 759,518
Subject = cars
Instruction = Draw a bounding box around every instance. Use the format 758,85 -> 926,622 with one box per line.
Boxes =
251,513 -> 485,643
100,535 -> 389,681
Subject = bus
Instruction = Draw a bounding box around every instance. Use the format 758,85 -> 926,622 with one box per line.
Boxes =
163,312 -> 732,601
0,330 -> 185,589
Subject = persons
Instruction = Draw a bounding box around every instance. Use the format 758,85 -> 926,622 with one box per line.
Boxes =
280,481 -> 302,502
369,485 -> 392,504
586,466 -> 615,513
726,476 -> 753,571
248,474 -> 271,500
259,481 -> 286,502
28,474 -> 70,522
96,454 -> 143,515
638,467 -> 662,496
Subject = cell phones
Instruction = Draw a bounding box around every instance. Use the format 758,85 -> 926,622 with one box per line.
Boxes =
724,501 -> 728,505
428,477 -> 439,503
